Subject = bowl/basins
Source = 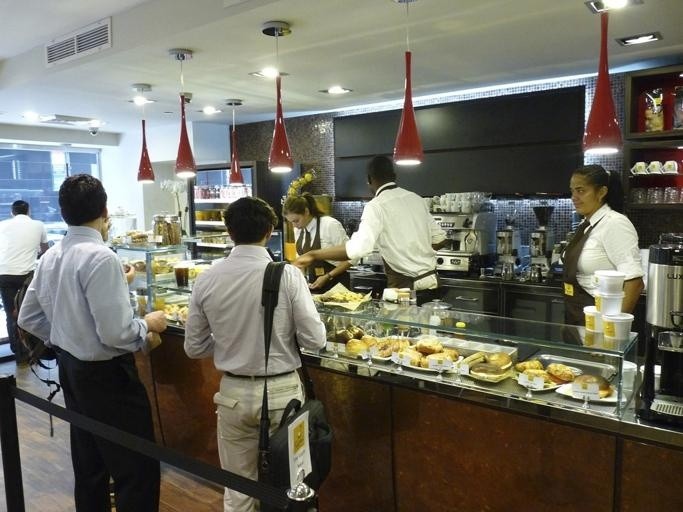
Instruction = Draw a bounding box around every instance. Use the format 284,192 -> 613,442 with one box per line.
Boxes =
194,209 -> 225,222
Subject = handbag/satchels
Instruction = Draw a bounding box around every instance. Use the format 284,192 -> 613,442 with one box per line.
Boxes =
257,399 -> 333,512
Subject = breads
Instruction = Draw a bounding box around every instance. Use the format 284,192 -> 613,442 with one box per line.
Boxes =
346,335 -> 612,397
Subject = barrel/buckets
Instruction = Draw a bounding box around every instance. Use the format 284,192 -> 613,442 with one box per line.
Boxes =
647,234 -> 683,352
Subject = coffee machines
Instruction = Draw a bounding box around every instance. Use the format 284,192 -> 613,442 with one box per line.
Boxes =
632,232 -> 683,431
434,212 -> 499,275
522,198 -> 555,281
491,196 -> 522,281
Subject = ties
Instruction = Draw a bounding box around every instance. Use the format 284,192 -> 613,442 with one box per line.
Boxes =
569,221 -> 589,248
303,228 -> 310,255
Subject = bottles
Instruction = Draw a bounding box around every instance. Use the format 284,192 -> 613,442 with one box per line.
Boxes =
189,233 -> 234,261
193,184 -> 252,201
152,215 -> 183,248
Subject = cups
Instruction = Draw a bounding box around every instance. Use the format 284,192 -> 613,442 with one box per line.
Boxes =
629,161 -> 677,177
629,187 -> 683,203
424,190 -> 490,213
396,287 -> 410,302
172,265 -> 189,287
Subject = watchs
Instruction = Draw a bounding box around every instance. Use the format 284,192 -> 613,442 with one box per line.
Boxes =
326,272 -> 334,282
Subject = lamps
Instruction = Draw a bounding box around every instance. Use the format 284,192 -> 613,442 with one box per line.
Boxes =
225,99 -> 245,185
259,20 -> 296,175
166,49 -> 199,180
579,2 -> 623,157
392,0 -> 425,168
134,83 -> 155,182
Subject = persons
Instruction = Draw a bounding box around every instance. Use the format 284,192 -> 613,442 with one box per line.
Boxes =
289,151 -> 449,307
0,199 -> 51,369
182,195 -> 331,512
559,162 -> 645,348
16,171 -> 170,512
279,192 -> 351,296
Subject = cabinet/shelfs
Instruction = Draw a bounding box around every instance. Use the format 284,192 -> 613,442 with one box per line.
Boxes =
622,64 -> 682,209
98,241 -> 185,460
185,162 -> 285,262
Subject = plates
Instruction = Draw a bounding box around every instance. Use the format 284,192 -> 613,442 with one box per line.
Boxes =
394,353 -> 460,374
335,348 -> 371,361
515,374 -> 555,395
562,383 -> 630,407
463,364 -> 512,386
371,349 -> 393,363
